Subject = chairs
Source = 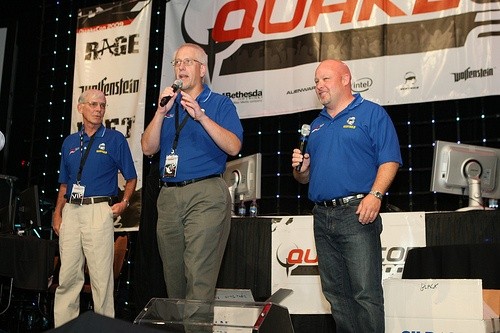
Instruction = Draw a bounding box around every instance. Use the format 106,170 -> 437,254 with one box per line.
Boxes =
51,232 -> 128,293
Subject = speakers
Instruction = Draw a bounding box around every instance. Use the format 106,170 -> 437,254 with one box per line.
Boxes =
131,298 -> 295,333
40,309 -> 170,333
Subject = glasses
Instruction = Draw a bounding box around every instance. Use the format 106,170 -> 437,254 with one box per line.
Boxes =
170,58 -> 202,67
83,101 -> 109,110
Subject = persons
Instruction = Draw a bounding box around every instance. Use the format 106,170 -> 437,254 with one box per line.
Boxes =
51,89 -> 137,328
140,43 -> 246,333
292,59 -> 402,333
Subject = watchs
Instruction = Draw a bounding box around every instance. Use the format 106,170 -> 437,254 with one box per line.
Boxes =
369,190 -> 383,199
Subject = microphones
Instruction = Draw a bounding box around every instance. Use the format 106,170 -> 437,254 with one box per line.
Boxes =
159,80 -> 183,107
296,124 -> 311,171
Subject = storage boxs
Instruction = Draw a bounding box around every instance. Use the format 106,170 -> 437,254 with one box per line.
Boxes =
213,288 -> 292,333
382,278 -> 500,333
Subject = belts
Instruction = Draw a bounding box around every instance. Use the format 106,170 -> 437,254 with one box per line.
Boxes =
315,193 -> 368,207
66,196 -> 107,206
161,173 -> 220,188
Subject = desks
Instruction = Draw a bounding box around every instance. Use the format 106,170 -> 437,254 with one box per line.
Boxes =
129,209 -> 500,332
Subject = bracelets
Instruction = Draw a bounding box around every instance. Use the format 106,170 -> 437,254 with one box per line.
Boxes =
121,199 -> 130,208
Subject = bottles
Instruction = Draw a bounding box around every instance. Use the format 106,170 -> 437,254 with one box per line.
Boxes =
489,198 -> 498,208
249,199 -> 257,217
238,202 -> 246,216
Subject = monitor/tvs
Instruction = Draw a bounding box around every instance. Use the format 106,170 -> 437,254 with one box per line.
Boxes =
429,140 -> 500,212
221,152 -> 261,217
